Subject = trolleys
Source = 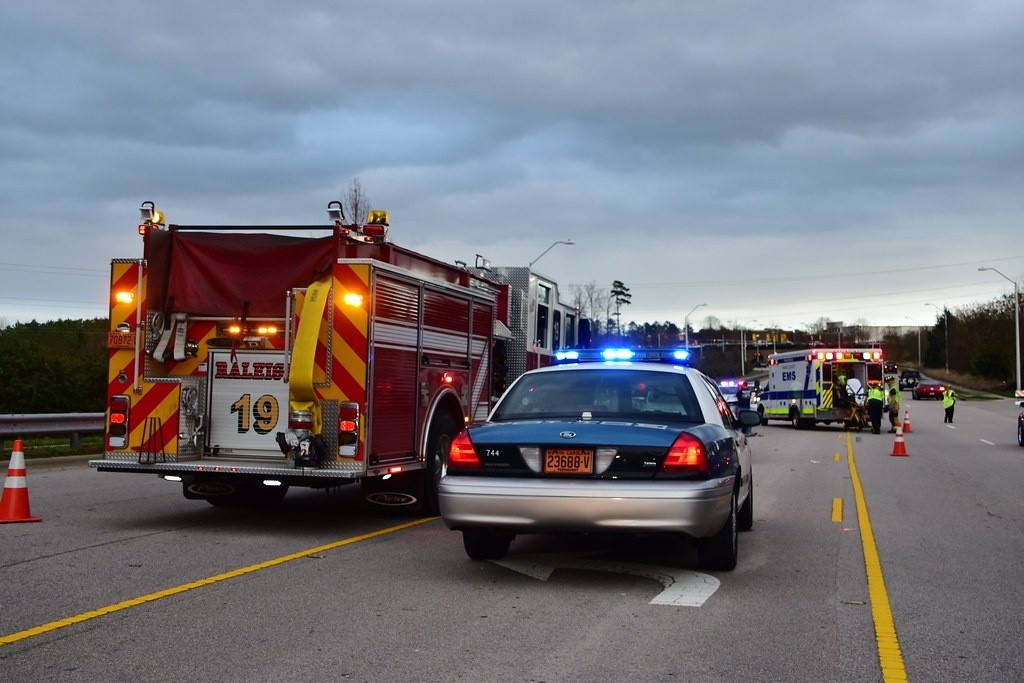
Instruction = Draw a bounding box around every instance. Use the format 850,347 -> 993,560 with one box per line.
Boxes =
841,395 -> 866,430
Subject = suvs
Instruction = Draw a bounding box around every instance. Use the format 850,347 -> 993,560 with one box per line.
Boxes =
899,369 -> 922,392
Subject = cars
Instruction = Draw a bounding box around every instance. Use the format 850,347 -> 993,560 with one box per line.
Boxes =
717,380 -> 763,422
436,346 -> 763,572
884,361 -> 899,375
912,377 -> 945,401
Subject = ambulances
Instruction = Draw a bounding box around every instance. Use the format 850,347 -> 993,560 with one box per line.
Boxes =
757,348 -> 884,431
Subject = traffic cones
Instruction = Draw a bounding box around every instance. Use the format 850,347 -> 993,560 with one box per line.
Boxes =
889,422 -> 909,457
1,438 -> 41,524
903,410 -> 911,433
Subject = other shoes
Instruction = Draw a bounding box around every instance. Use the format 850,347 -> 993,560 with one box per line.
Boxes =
872,430 -> 880,434
887,430 -> 896,433
945,421 -> 947,423
948,420 -> 952,423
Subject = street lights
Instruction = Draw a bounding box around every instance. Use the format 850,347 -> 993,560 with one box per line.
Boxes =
925,302 -> 950,374
684,302 -> 708,351
903,315 -> 921,367
976,266 -> 1021,392
741,318 -> 757,377
800,323 -> 815,348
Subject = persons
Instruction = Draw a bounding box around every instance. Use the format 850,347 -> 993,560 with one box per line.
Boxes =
941,384 -> 959,423
833,373 -> 902,435
736,378 -> 769,437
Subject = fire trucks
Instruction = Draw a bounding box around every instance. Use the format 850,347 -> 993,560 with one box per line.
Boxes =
87,199 -> 593,523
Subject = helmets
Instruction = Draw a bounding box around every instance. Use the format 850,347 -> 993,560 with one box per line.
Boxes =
884,375 -> 896,382
